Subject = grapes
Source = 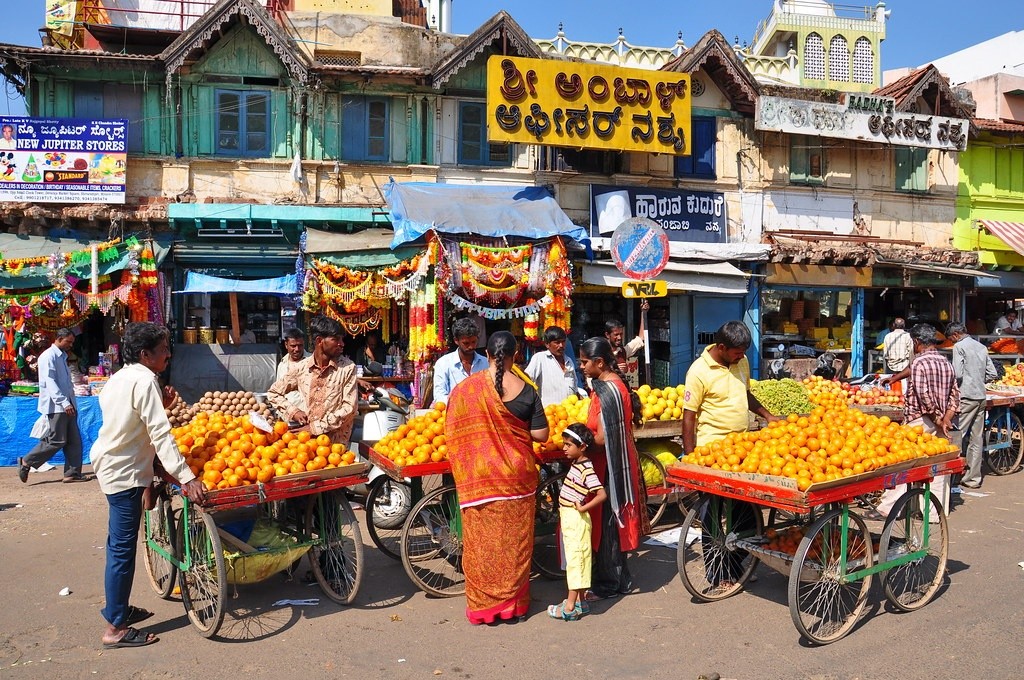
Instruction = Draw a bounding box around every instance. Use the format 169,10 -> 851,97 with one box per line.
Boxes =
749,377 -> 816,416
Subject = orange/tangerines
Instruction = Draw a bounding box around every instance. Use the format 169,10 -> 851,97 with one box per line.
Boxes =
682,393 -> 958,491
632,384 -> 686,421
374,394 -> 591,467
169,411 -> 356,491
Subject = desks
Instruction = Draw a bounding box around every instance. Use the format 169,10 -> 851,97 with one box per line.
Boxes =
0,395 -> 101,469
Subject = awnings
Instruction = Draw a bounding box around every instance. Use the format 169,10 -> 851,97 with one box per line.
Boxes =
0,120 -> 772,297
976,218 -> 1024,257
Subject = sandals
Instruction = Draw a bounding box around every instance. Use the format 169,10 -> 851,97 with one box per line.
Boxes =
546,580 -> 632,620
858,509 -> 901,521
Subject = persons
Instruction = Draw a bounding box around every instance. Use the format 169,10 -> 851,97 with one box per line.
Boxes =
547,424 -> 607,623
446,330 -> 550,629
864,300 -> 1010,359
269,326 -> 315,422
580,337 -> 652,602
880,338 -> 944,386
856,323 -> 960,525
17,327 -> 93,484
229,294 -> 658,409
267,314 -> 359,596
983,308 -> 1024,349
883,317 -> 914,374
682,319 -> 787,593
943,321 -> 998,488
89,323 -> 210,653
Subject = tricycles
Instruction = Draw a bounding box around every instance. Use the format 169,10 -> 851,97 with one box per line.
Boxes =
364,445 -> 668,599
678,479 -> 950,647
139,456 -> 364,638
949,406 -> 1024,477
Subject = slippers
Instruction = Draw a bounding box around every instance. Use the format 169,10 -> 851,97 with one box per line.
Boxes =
126,605 -> 153,628
102,626 -> 158,650
66,475 -> 92,482
18,456 -> 30,483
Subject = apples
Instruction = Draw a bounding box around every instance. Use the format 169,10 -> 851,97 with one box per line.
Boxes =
801,374 -> 906,406
999,362 -> 1024,387
